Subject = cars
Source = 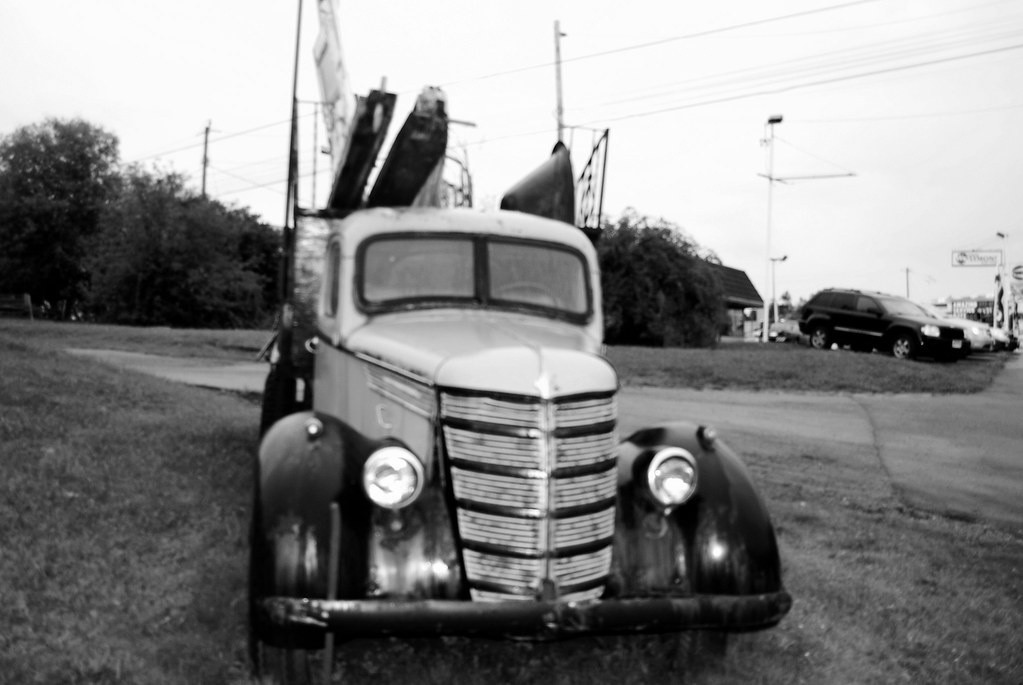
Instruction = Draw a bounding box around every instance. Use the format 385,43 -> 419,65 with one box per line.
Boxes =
766,294 -> 1012,353
246,199 -> 799,685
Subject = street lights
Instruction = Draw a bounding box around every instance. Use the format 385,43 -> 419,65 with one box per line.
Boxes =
765,166 -> 862,302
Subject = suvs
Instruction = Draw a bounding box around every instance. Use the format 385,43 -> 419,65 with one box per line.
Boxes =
798,286 -> 968,364
